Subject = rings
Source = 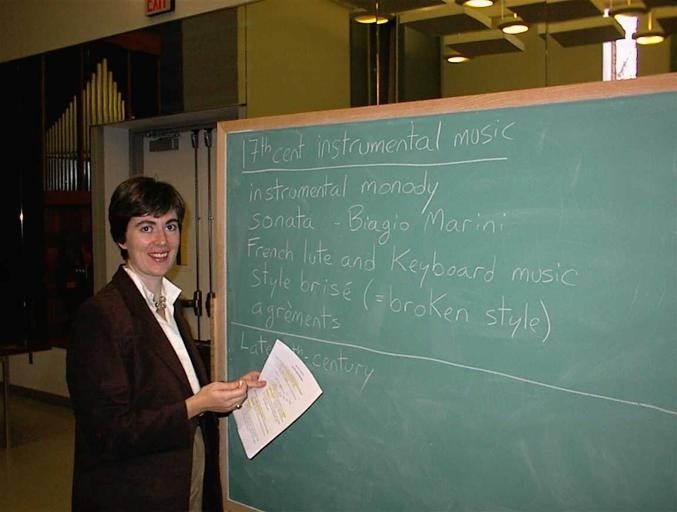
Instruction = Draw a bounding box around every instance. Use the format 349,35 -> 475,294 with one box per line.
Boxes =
235,404 -> 242,409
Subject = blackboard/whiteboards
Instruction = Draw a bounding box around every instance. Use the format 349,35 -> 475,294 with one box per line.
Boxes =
212,73 -> 677,512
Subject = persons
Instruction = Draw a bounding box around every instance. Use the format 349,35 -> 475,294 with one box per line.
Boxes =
65,176 -> 265,512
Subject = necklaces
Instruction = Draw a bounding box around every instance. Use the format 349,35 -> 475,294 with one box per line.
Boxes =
152,294 -> 167,313
